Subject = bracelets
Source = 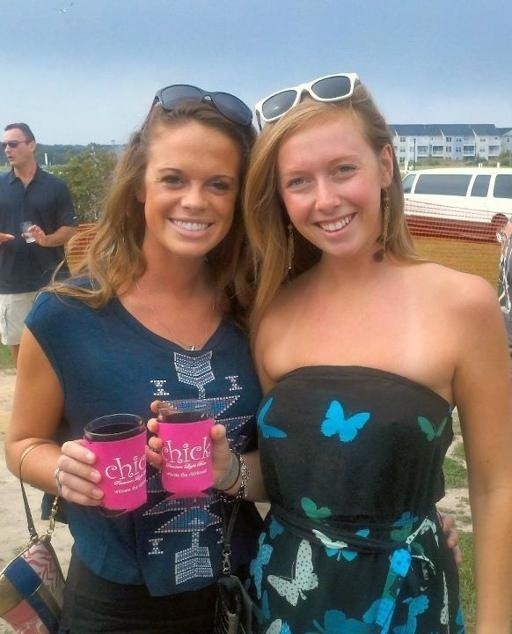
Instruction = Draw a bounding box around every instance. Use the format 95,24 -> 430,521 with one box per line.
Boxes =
217,451 -> 251,501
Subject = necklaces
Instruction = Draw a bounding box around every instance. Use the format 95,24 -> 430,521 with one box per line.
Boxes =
132,277 -> 223,352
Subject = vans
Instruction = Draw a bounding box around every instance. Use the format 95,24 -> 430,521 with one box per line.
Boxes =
396,164 -> 511,227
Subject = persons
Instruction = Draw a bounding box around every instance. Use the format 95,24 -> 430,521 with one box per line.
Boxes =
1,121 -> 81,363
146,70 -> 512,633
4,83 -> 264,634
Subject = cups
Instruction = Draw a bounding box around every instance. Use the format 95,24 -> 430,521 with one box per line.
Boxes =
20,220 -> 37,243
157,402 -> 217,494
81,412 -> 148,516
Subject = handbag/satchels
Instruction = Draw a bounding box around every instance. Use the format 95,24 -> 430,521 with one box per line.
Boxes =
0,531 -> 70,634
212,573 -> 255,634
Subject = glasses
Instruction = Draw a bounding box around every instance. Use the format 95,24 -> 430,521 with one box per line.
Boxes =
252,68 -> 366,135
1,140 -> 30,149
148,82 -> 258,129
